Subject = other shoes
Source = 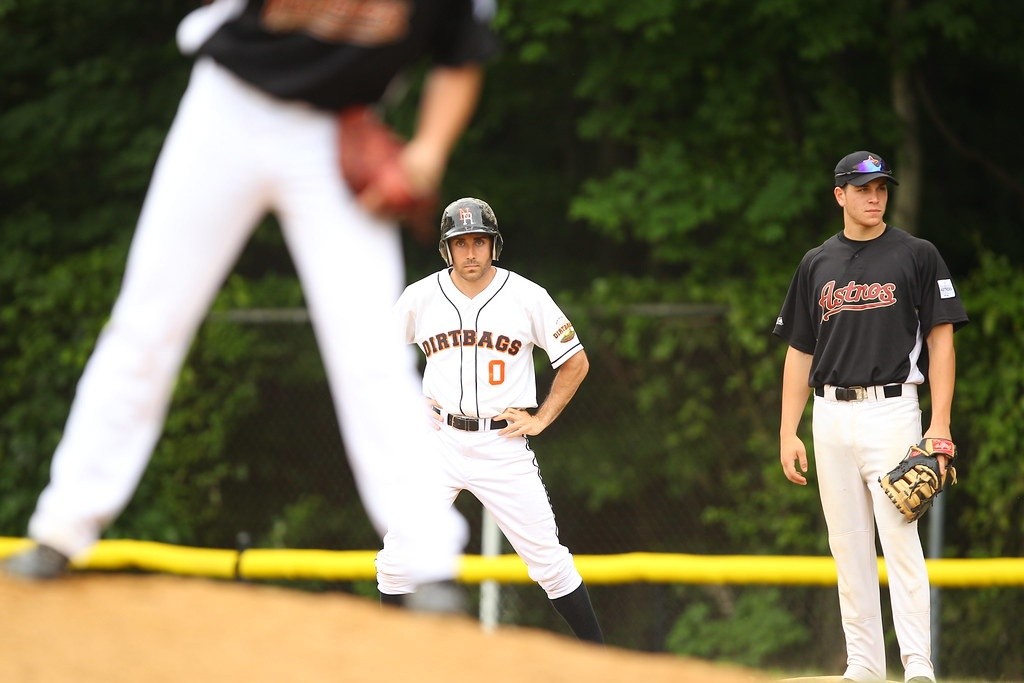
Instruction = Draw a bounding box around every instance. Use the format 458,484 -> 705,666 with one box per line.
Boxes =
410,582 -> 463,614
5,543 -> 70,580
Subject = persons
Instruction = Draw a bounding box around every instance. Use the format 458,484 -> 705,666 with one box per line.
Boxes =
393,196 -> 603,645
14,0 -> 500,616
771,150 -> 969,682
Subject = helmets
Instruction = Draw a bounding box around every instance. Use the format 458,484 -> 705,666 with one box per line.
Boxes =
438,197 -> 503,267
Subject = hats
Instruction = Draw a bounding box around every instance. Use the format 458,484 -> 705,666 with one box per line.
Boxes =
834,151 -> 899,188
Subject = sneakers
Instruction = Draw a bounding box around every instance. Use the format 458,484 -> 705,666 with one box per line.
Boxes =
905,662 -> 936,683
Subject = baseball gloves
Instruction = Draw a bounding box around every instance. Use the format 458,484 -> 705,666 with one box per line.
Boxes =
878,437 -> 958,527
261,0 -> 417,46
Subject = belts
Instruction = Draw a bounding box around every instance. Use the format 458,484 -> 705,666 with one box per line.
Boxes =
432,406 -> 508,432
815,385 -> 902,402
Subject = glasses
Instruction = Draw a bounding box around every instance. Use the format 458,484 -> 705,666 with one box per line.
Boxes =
835,159 -> 892,178
